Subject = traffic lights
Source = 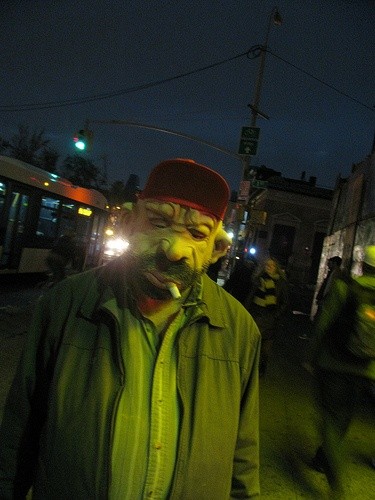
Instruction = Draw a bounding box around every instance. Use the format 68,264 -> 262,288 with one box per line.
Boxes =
249,165 -> 277,182
73,129 -> 88,152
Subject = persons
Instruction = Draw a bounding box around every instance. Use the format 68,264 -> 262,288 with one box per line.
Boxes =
298,256 -> 343,340
207,258 -> 221,283
310,246 -> 375,500
246,256 -> 291,377
0,158 -> 260,500
45,230 -> 82,284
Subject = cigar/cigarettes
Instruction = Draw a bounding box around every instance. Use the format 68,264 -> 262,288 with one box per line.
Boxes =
167,281 -> 181,299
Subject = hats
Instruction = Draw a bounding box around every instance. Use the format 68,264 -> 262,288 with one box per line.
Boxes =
137,156 -> 231,220
363,245 -> 375,268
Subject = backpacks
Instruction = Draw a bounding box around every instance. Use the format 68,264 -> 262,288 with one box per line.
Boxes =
336,272 -> 375,365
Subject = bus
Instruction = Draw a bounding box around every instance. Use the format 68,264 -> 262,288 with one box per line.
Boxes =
0,155 -> 110,280
